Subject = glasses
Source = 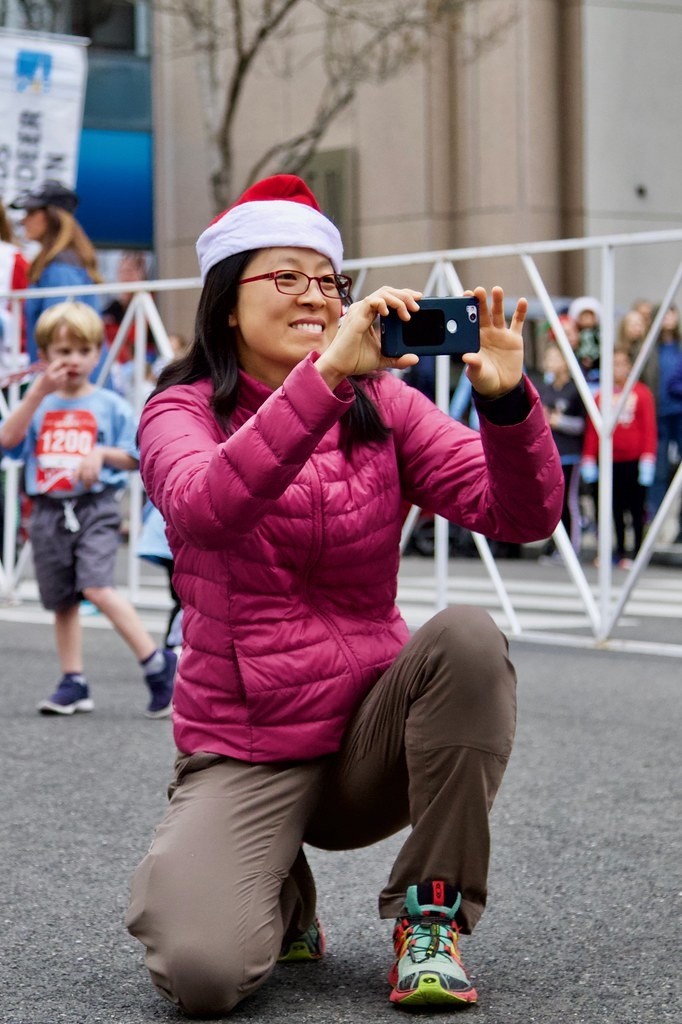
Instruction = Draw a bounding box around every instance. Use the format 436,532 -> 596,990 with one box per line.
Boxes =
237,270 -> 352,299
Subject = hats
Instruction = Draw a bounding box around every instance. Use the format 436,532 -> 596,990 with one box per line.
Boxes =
196,175 -> 343,279
568,296 -> 599,327
9,180 -> 77,212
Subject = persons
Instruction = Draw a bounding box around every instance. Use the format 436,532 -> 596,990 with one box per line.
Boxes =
0,178 -> 682,713
123,170 -> 564,1012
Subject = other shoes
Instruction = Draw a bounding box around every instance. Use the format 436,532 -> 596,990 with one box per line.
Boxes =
619,557 -> 635,569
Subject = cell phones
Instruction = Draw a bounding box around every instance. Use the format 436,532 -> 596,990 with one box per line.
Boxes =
379,296 -> 480,358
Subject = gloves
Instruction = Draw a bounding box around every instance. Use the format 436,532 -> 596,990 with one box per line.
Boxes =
579,457 -> 599,483
637,459 -> 656,486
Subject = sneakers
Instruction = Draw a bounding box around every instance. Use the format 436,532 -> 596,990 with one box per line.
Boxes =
390,879 -> 478,1006
38,678 -> 95,714
274,912 -> 325,962
145,650 -> 181,718
